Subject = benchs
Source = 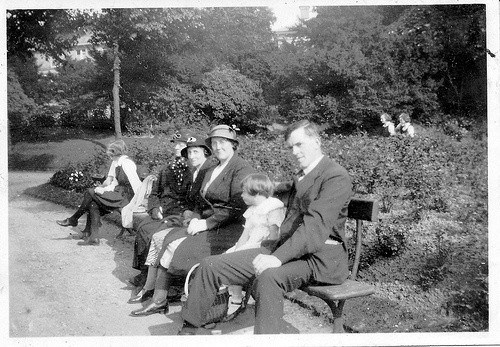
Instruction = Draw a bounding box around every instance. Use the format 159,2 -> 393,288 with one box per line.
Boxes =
239,197 -> 379,334
91,176 -> 146,239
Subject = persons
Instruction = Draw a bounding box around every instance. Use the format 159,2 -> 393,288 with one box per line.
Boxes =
57,139 -> 136,246
128,124 -> 257,318
129,137 -> 210,290
174,119 -> 354,334
377,112 -> 395,138
215,171 -> 287,322
395,113 -> 415,137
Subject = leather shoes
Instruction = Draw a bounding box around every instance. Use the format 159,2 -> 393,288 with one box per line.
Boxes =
57,218 -> 75,226
132,300 -> 168,315
129,288 -> 154,303
78,238 -> 99,245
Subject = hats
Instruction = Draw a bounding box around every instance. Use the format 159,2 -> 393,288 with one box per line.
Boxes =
181,136 -> 212,156
204,124 -> 239,145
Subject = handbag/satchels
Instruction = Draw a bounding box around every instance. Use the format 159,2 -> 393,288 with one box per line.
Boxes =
180,262 -> 229,323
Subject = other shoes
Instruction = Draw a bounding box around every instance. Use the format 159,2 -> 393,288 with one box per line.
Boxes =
178,323 -> 195,334
129,274 -> 146,287
